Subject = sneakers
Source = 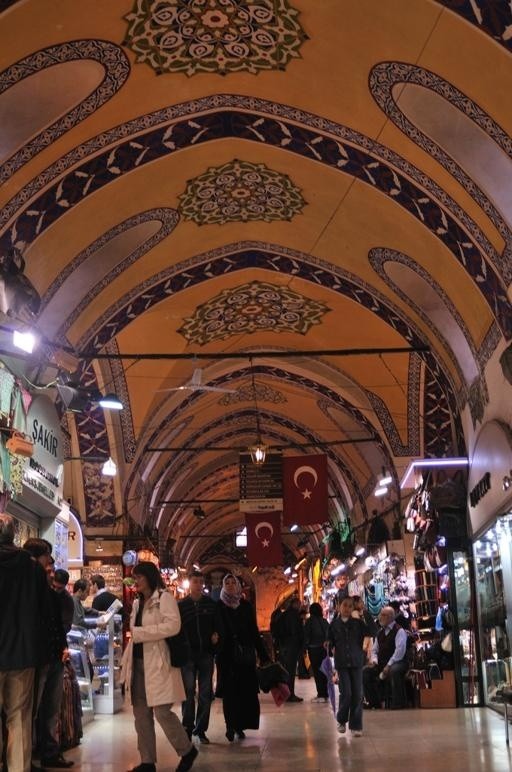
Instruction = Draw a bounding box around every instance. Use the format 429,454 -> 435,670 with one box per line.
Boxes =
351,729 -> 363,737
336,721 -> 346,734
287,695 -> 303,702
41,755 -> 73,768
127,764 -> 156,772
175,745 -> 198,772
225,730 -> 245,742
311,697 -> 329,703
198,734 -> 209,744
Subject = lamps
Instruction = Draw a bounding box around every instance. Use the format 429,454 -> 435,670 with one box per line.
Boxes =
82,394 -> 123,409
248,356 -> 268,466
6,431 -> 34,456
56,367 -> 90,414
193,501 -> 205,519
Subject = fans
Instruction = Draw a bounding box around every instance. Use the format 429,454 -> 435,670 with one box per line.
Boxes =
149,357 -> 238,394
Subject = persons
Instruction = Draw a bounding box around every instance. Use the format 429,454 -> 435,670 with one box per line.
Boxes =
323,598 -> 377,737
120,561 -> 201,771
166,571 -> 220,747
1,512 -> 124,772
210,573 -> 273,741
269,593 -> 411,716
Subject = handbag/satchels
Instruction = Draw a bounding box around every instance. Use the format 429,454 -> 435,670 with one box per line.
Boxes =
404,490 -> 454,689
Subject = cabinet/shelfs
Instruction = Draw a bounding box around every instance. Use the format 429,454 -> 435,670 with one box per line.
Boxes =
91,616 -> 123,714
420,670 -> 456,708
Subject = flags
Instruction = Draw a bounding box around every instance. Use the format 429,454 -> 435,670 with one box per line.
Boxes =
244,510 -> 284,568
282,453 -> 330,528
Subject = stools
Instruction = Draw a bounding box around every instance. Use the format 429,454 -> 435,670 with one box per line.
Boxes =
379,677 -> 409,710
